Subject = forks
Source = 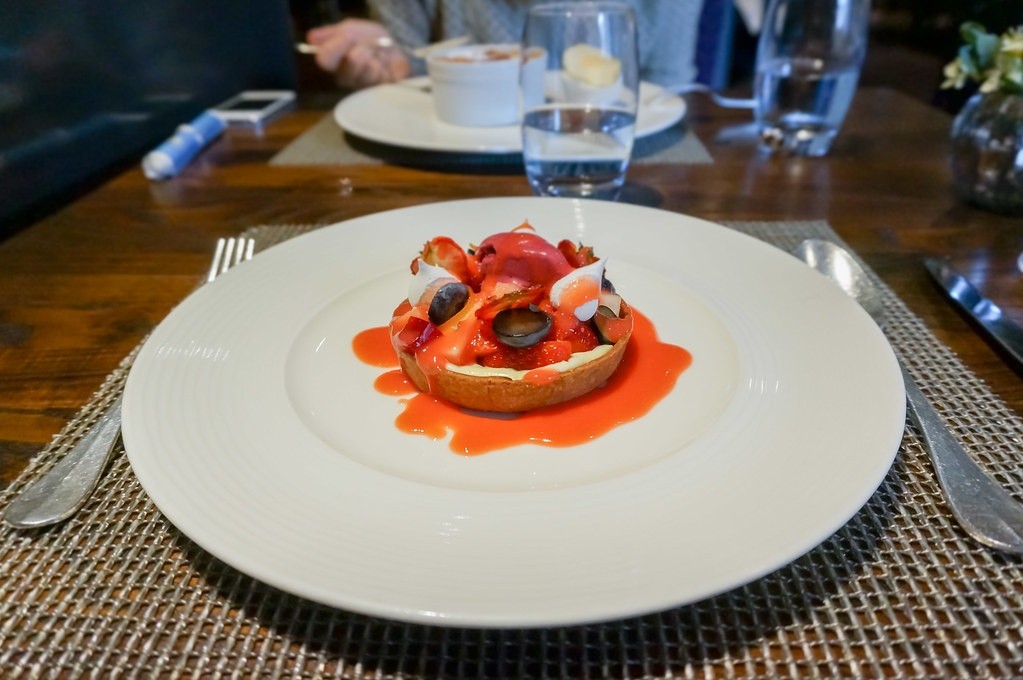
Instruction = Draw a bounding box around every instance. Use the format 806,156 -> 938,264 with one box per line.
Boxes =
3,238 -> 256,529
648,82 -> 754,110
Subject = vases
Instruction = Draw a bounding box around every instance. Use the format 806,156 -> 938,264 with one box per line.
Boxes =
947,85 -> 1022,216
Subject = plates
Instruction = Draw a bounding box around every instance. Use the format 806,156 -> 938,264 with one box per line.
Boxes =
332,68 -> 688,156
119,196 -> 908,630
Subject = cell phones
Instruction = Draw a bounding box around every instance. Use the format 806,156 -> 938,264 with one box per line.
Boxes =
208,91 -> 298,125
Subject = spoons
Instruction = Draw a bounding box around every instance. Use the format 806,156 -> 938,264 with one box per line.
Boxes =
297,34 -> 472,59
793,238 -> 1023,557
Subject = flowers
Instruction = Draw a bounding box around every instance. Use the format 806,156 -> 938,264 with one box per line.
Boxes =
941,22 -> 1022,89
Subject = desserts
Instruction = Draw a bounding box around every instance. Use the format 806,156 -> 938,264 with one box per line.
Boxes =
387,231 -> 635,411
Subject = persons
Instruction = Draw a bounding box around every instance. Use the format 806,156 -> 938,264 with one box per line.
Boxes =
307,0 -> 705,90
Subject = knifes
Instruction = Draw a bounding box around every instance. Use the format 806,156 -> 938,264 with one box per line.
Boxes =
917,255 -> 1023,362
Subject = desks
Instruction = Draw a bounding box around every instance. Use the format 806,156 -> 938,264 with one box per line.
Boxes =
0,84 -> 1022,680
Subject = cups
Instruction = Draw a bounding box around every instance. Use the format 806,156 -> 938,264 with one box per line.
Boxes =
753,0 -> 871,156
559,70 -> 624,107
425,43 -> 548,126
519,0 -> 640,201
